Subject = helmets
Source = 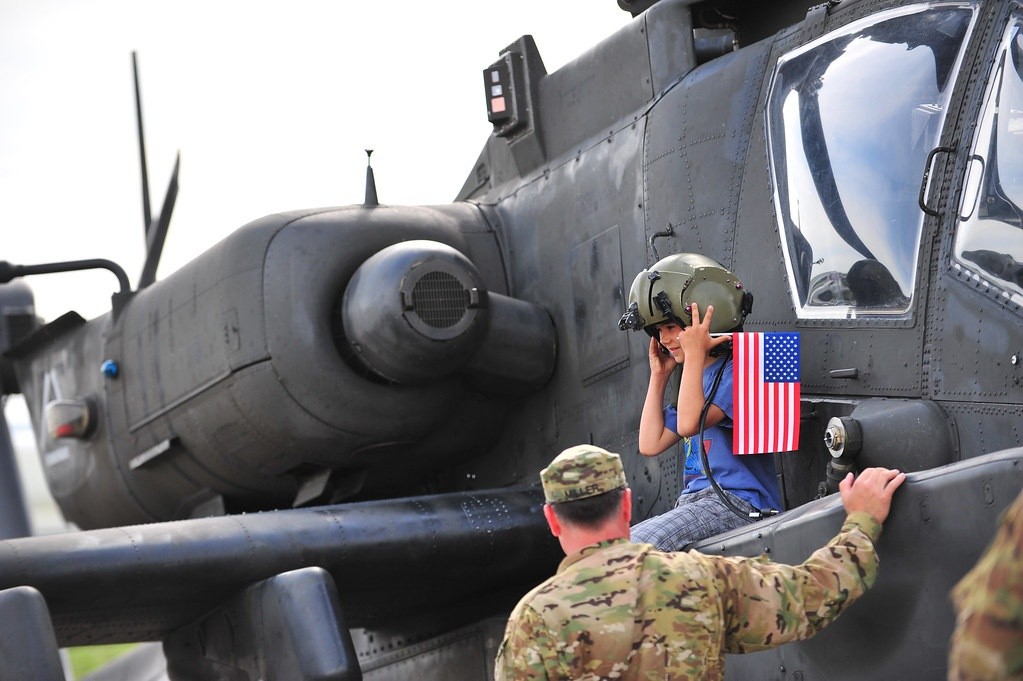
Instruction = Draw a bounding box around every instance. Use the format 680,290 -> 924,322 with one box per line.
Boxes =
618,253 -> 753,354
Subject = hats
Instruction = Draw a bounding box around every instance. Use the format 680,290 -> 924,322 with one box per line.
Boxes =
540,444 -> 626,502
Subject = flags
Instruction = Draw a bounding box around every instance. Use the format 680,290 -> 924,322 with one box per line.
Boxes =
732,332 -> 801,456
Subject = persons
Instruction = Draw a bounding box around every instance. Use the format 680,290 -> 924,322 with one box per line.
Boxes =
493,443 -> 907,681
617,251 -> 785,552
945,488 -> 1023,681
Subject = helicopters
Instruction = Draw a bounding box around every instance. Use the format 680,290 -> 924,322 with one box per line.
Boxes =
4,2 -> 1023,680
